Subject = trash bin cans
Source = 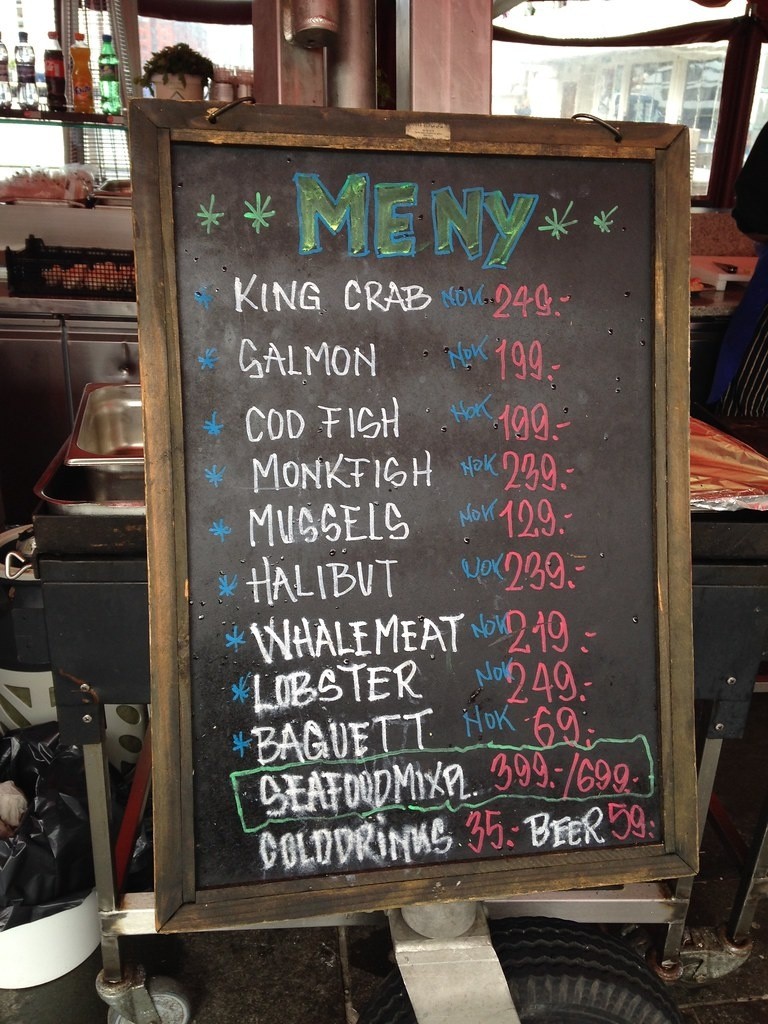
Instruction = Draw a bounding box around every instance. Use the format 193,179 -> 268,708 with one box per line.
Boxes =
0,520 -> 148,805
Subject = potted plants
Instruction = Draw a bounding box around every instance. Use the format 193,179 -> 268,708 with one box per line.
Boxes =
134,43 -> 213,100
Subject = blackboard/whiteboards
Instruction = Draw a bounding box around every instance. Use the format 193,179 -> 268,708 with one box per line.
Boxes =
121,94 -> 703,938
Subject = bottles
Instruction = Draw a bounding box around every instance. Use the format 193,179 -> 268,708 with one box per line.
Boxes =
69,33 -> 96,115
14,31 -> 40,111
98,35 -> 123,116
0,31 -> 12,110
43,31 -> 67,112
